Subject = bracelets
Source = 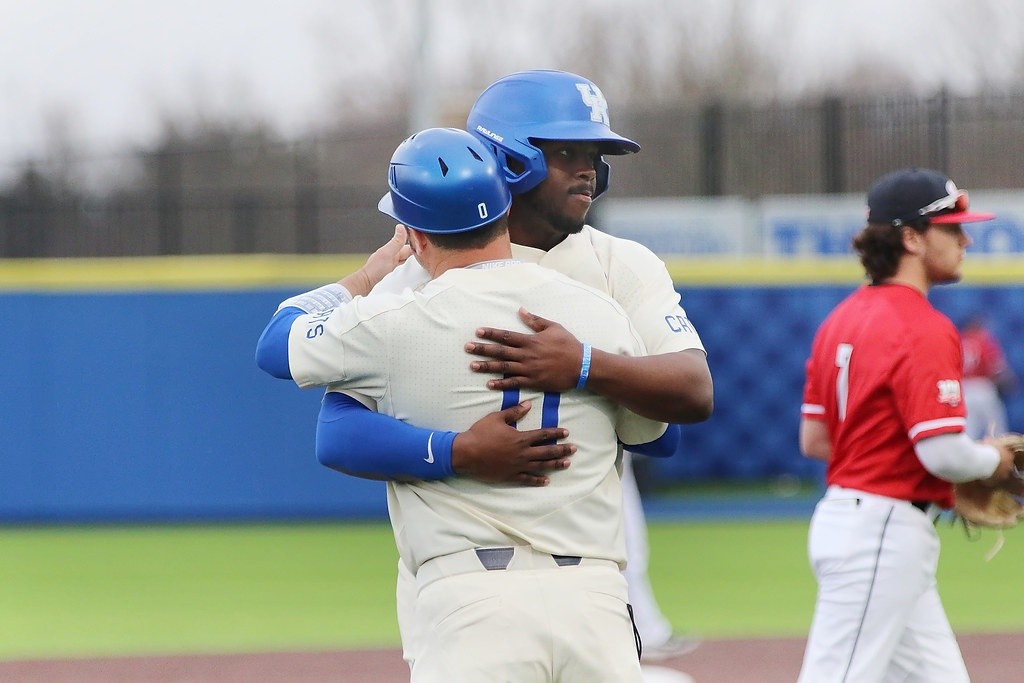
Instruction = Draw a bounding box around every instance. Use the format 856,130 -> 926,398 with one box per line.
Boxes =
575,342 -> 592,393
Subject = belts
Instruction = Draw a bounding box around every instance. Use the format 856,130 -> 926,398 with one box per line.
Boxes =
912,499 -> 931,513
475,548 -> 582,570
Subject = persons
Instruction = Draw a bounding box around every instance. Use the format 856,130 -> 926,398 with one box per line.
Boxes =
255,126 -> 683,682
794,165 -> 1014,682
961,310 -> 1013,442
314,69 -> 714,682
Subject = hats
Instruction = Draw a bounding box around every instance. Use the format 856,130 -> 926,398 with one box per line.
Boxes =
867,167 -> 997,228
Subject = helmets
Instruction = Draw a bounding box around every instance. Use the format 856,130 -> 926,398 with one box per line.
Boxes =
466,69 -> 641,202
377,128 -> 512,234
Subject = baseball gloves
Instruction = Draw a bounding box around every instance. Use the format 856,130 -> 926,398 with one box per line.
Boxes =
939,430 -> 1023,541
